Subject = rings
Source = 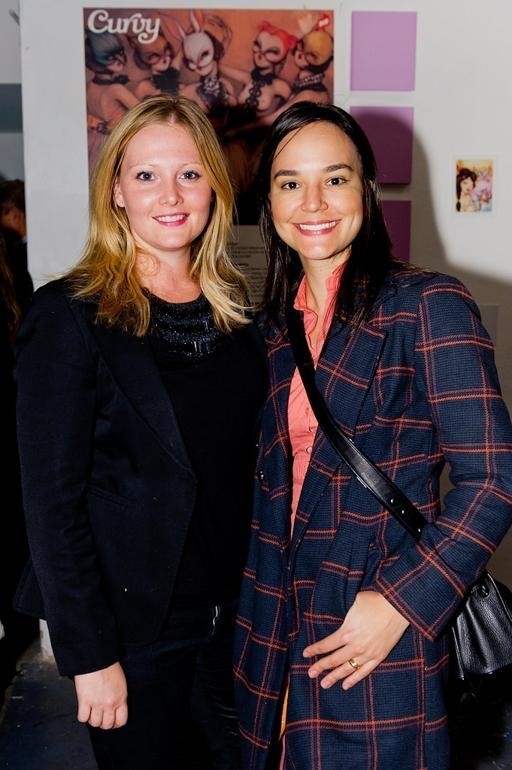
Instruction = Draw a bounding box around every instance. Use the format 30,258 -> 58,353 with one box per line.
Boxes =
348,657 -> 361,671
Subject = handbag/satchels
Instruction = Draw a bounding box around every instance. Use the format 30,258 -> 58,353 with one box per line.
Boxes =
282,298 -> 512,704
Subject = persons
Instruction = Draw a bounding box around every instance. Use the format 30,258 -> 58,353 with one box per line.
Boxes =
15,94 -> 269,768
218,102 -> 512,768
0,172 -> 34,351
